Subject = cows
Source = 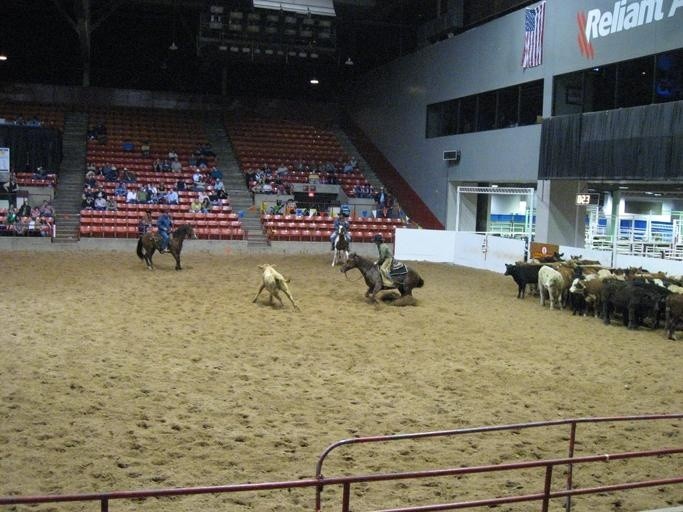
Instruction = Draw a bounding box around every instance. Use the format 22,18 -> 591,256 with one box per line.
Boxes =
251,263 -> 300,312
504,250 -> 683,340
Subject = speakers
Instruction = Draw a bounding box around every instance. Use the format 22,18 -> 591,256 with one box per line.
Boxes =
443,150 -> 457,161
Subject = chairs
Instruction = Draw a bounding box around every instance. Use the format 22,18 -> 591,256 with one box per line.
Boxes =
0,168 -> 56,234
77,102 -> 407,244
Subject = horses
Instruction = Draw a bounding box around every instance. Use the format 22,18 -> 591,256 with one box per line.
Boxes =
339,251 -> 424,305
136,222 -> 198,270
330,223 -> 350,267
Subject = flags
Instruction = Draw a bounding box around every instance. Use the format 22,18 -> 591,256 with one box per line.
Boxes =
520,1 -> 545,68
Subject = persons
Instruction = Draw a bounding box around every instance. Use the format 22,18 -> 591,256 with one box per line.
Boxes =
246,155 -> 394,218
82,121 -> 228,213
157,206 -> 174,254
330,209 -> 352,252
373,234 -> 408,290
1,166 -> 56,237
139,220 -> 150,240
13,113 -> 59,131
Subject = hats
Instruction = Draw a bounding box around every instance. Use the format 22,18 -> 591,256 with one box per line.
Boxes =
372,234 -> 385,241
